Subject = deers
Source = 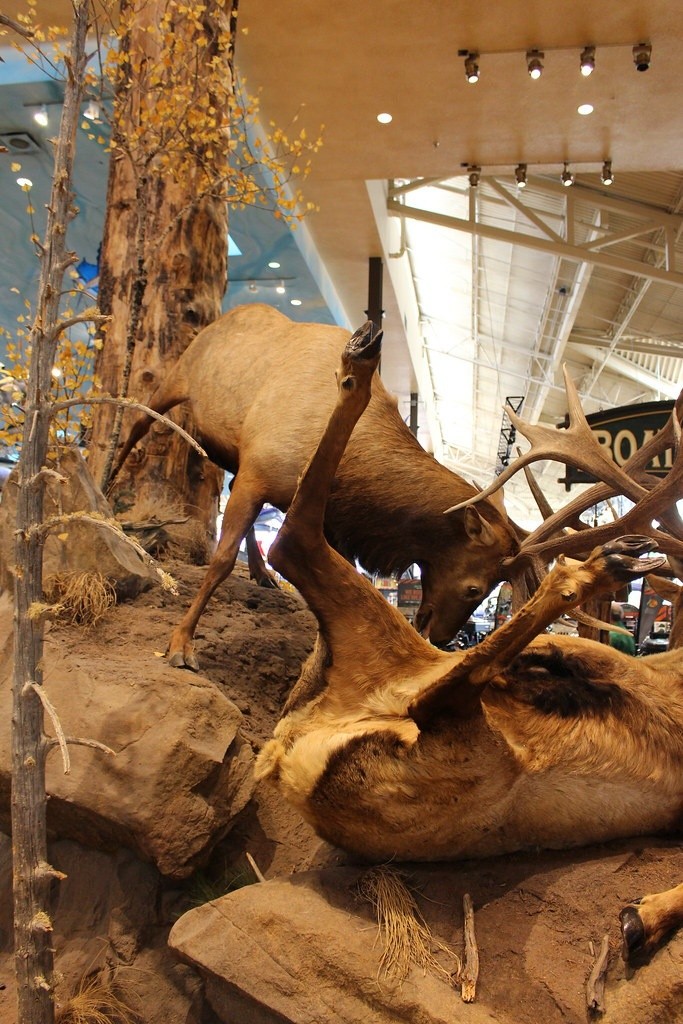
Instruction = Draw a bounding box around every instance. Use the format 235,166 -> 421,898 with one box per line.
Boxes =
103,300 -> 683,963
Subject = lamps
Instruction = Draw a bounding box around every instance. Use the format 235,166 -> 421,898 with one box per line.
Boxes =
275,279 -> 285,294
464,53 -> 479,83
561,163 -> 572,186
526,50 -> 544,79
515,164 -> 527,188
632,44 -> 652,71
83,98 -> 100,119
580,47 -> 595,77
468,166 -> 482,188
34,103 -> 48,127
601,161 -> 614,185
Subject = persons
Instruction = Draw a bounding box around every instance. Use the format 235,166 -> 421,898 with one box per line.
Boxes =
609,605 -> 636,656
